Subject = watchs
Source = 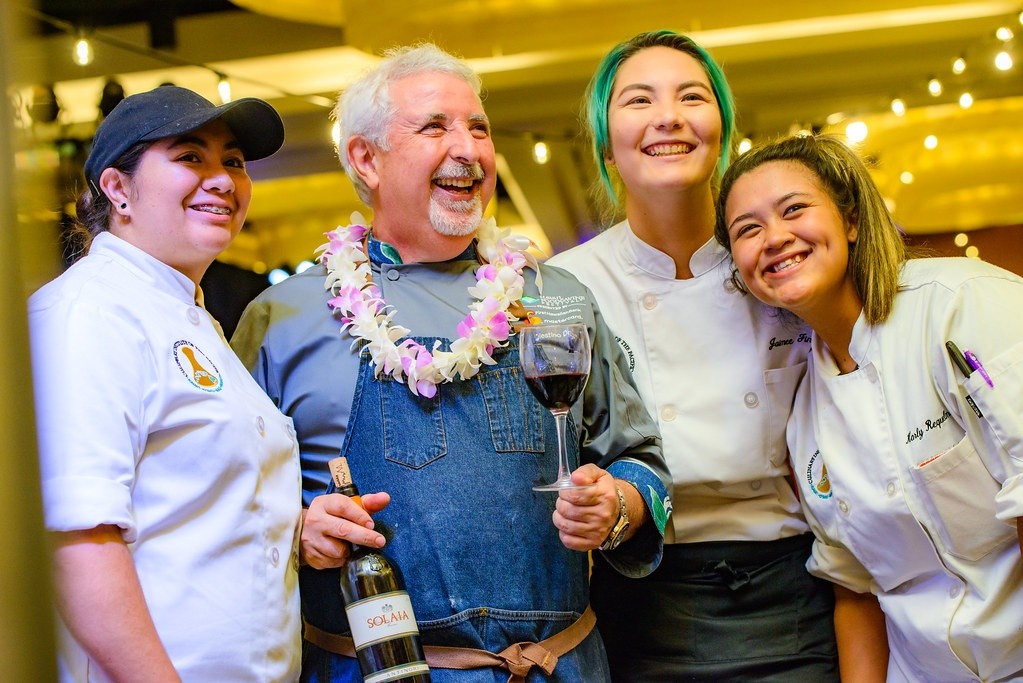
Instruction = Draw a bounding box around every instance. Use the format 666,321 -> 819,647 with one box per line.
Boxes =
598,486 -> 630,550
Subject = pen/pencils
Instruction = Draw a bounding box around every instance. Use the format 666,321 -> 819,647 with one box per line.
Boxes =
946,341 -> 994,388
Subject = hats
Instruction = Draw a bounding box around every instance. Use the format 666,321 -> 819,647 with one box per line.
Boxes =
84,85 -> 284,209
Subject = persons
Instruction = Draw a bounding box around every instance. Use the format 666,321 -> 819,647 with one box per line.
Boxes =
231,45 -> 675,683
715,137 -> 1023,683
536,30 -> 844,683
27,87 -> 305,683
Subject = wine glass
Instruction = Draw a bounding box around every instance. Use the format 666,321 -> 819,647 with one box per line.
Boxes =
518,323 -> 599,491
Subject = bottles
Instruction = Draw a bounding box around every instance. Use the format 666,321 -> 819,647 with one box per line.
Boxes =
327,456 -> 434,683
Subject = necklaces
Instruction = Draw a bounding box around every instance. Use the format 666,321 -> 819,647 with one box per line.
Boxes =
313,215 -> 541,397
